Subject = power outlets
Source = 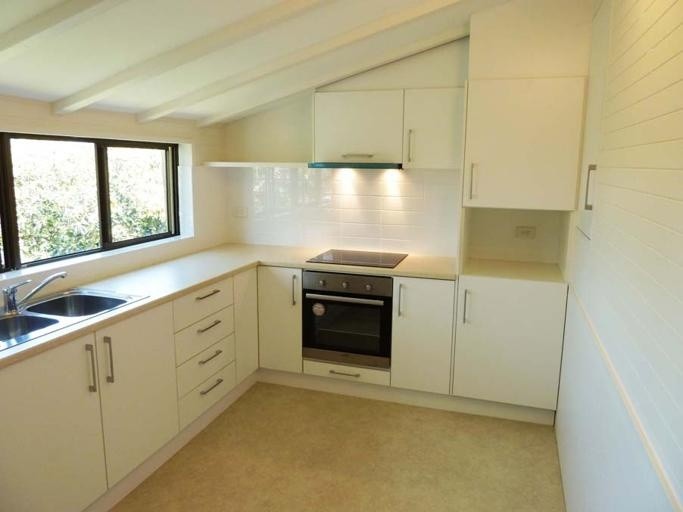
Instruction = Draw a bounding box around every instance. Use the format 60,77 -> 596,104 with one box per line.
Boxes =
515,226 -> 536,241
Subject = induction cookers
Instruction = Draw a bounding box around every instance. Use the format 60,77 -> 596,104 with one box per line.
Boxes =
306,248 -> 408,268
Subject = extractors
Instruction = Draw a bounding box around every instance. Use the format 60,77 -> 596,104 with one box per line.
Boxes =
307,162 -> 402,169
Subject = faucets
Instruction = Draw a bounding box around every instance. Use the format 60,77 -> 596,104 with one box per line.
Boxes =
1,270 -> 68,315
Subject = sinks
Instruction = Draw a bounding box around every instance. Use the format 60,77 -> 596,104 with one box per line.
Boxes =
21,285 -> 151,319
0,309 -> 61,355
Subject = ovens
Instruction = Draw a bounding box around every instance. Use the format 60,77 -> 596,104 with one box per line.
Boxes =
303,271 -> 393,370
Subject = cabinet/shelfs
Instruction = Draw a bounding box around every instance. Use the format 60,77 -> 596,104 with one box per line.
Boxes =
312,90 -> 404,169
463,77 -> 588,211
173,275 -> 234,455
234,268 -> 258,402
449,260 -> 568,426
258,265 -> 304,388
302,359 -> 391,401
403,87 -> 467,170
390,276 -> 456,413
575,1 -> 610,240
0,300 -> 174,512
